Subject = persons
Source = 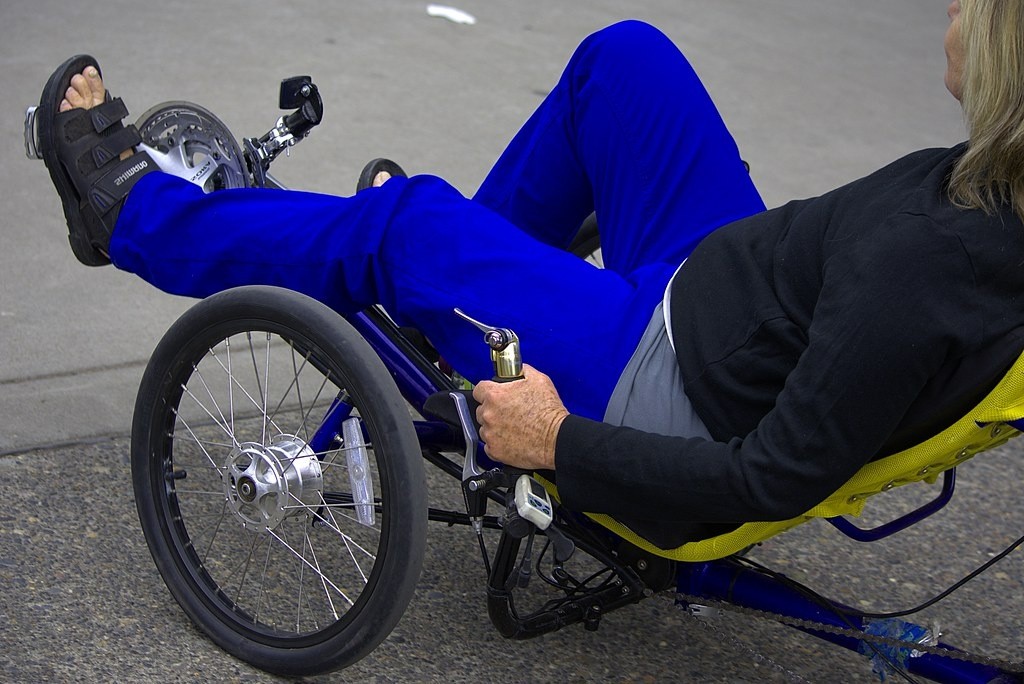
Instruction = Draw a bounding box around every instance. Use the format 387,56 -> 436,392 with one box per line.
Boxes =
37,0 -> 1024,551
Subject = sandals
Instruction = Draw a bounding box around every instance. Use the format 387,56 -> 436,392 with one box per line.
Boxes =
39,54 -> 163,267
356,158 -> 441,364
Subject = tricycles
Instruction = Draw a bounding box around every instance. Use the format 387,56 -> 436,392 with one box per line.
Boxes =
24,75 -> 1024,684
